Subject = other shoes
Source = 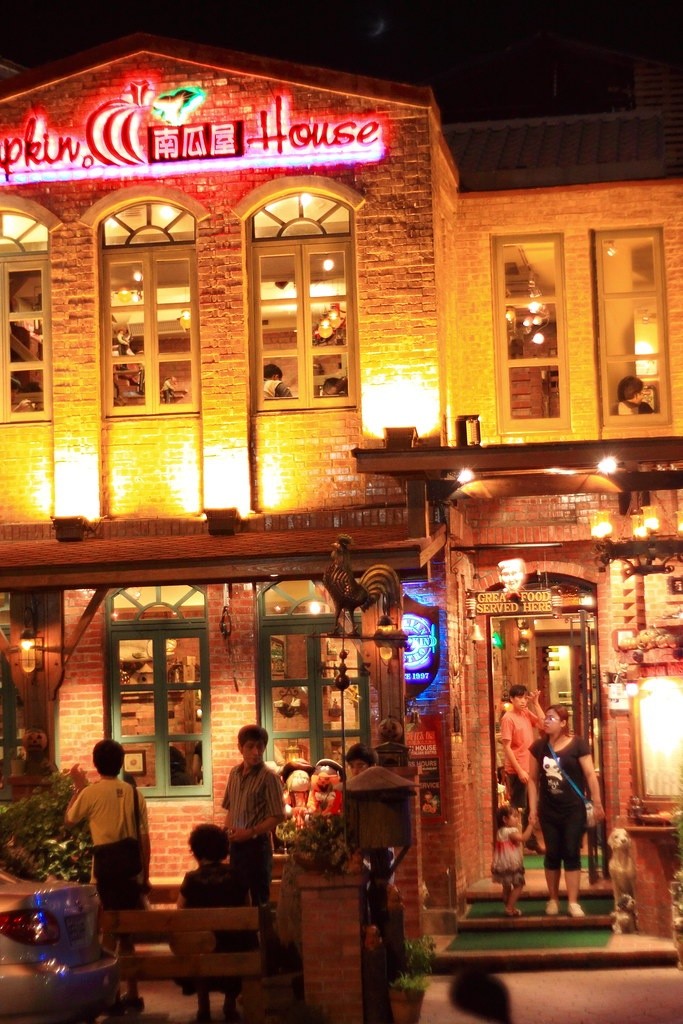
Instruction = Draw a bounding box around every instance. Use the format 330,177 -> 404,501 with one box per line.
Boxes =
120,993 -> 145,1010
504,909 -> 522,917
523,848 -> 537,855
535,846 -> 546,854
104,1002 -> 126,1016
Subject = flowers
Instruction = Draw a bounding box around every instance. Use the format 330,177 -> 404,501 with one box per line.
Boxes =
275,783 -> 354,864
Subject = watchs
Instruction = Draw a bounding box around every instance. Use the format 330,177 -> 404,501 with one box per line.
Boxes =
251,825 -> 257,839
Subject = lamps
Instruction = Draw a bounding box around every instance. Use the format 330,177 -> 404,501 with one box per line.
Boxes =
589,489 -> 683,577
50,515 -> 93,542
19,596 -> 36,673
506,289 -> 550,345
134,290 -> 142,301
318,304 -> 345,338
377,592 -> 395,661
202,508 -> 242,537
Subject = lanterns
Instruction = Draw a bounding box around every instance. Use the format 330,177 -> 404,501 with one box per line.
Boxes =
23,728 -> 47,752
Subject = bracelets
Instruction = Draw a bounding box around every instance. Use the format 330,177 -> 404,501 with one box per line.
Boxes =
72,788 -> 81,794
594,804 -> 602,810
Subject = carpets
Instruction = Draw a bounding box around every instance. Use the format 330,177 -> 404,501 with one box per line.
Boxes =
465,898 -> 614,917
444,928 -> 612,951
522,854 -> 603,869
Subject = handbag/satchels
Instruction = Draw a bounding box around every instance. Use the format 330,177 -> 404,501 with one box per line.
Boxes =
584,801 -> 595,827
90,836 -> 143,881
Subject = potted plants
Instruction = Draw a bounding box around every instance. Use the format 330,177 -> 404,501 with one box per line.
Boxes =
389,935 -> 436,1024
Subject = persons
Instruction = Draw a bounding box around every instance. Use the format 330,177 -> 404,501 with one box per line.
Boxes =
345,742 -> 395,921
124,770 -> 137,787
263,363 -> 293,397
491,684 -> 606,917
170,824 -> 253,1024
321,378 -> 345,395
11,379 -> 43,412
169,740 -> 202,786
161,377 -> 175,404
116,323 -> 132,369
264,757 -> 347,832
610,375 -> 654,416
64,739 -> 151,1013
221,725 -> 287,948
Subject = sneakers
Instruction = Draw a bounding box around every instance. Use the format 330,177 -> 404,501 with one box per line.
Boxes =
566,904 -> 584,918
545,900 -> 561,915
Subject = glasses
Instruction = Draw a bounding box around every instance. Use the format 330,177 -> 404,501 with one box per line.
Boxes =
541,716 -> 561,722
346,761 -> 369,772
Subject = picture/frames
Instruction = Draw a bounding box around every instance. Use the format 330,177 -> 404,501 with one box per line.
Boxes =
271,637 -> 286,677
123,750 -> 147,776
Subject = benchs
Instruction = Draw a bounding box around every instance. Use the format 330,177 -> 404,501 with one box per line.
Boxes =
97,904 -> 274,1020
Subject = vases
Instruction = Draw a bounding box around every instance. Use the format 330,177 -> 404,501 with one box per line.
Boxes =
287,852 -> 342,875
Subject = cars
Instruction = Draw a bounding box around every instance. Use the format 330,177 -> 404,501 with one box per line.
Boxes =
0,868 -> 120,1024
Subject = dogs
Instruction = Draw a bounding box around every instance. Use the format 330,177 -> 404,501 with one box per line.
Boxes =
606,826 -> 635,932
